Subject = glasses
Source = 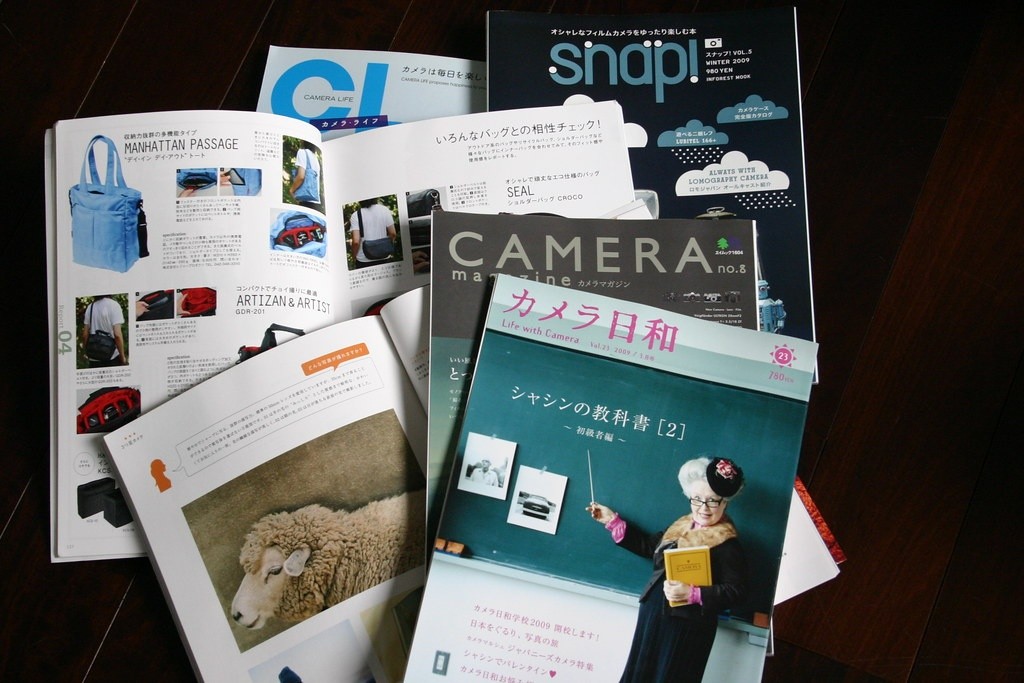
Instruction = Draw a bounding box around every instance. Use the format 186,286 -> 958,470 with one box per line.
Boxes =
689,496 -> 723,507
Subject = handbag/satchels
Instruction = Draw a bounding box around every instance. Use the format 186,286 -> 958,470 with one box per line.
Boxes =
291,149 -> 319,201
235,323 -> 305,365
180,287 -> 217,317
85,330 -> 117,361
230,168 -> 262,196
177,168 -> 217,191
362,237 -> 393,260
69,135 -> 150,272
407,189 -> 442,218
270,209 -> 327,259
136,289 -> 174,321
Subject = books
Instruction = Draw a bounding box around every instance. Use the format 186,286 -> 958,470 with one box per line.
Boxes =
43,2 -> 848,682
663,545 -> 711,607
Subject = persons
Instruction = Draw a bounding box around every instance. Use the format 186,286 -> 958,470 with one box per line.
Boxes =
136,302 -> 149,318
471,458 -> 499,486
82,295 -> 127,368
176,293 -> 191,315
349,197 -> 397,268
290,139 -> 322,210
587,457 -> 747,683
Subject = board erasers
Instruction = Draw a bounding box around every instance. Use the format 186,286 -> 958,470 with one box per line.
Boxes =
434,537 -> 465,555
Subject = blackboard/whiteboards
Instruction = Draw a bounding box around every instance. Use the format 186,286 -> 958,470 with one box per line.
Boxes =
434,328 -> 810,636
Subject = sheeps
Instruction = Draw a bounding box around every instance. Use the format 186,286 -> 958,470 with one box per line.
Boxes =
230,487 -> 425,630
